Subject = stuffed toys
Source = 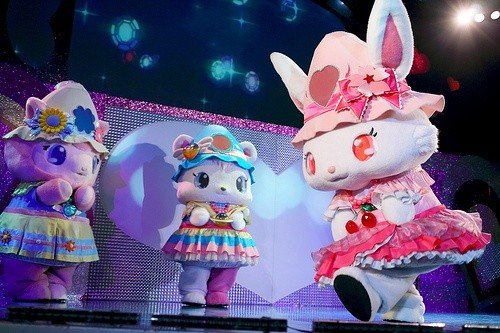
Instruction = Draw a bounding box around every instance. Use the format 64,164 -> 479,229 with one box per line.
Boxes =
0,80 -> 111,301
269,0 -> 491,322
161,124 -> 259,305
